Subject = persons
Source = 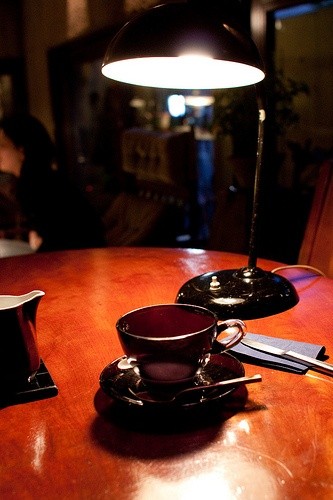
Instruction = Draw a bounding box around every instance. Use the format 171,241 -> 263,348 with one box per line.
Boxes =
0,114 -> 105,253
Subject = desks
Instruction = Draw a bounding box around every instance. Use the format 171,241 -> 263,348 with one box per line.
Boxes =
0,247 -> 333,500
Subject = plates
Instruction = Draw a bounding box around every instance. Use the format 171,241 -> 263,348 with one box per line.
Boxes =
99,350 -> 245,412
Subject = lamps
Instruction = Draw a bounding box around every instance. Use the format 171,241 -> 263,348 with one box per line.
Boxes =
100,0 -> 299,321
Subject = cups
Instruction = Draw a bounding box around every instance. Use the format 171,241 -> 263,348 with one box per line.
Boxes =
115,303 -> 247,391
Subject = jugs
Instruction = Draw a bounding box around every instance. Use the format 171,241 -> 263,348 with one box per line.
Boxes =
0,289 -> 46,390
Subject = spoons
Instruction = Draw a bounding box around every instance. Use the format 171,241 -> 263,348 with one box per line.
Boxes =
133,372 -> 263,403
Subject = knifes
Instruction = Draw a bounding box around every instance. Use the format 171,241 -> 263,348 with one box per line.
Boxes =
240,338 -> 333,376
